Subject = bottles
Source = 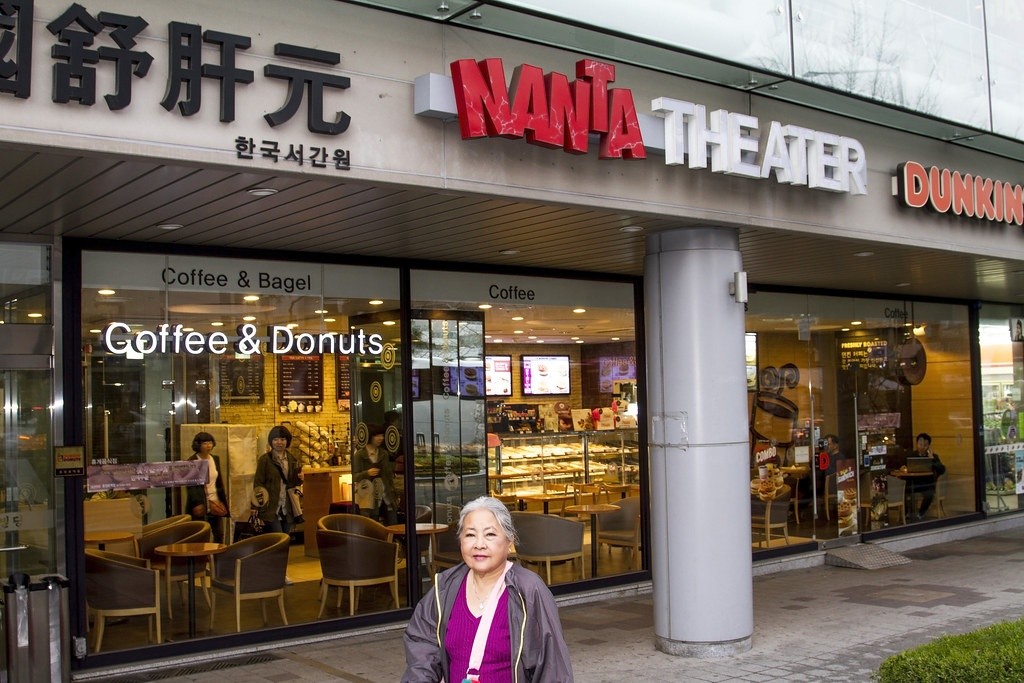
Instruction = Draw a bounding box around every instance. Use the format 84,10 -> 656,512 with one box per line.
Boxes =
344,447 -> 351,465
332,448 -> 342,465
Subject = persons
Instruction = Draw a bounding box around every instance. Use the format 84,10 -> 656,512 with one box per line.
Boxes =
788,434 -> 845,523
185,430 -> 232,544
354,411 -> 404,525
252,426 -> 304,532
903,433 -> 945,521
1015,320 -> 1024,341
401,497 -> 575,683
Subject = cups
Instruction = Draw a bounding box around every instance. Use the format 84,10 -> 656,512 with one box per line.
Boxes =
759,466 -> 768,481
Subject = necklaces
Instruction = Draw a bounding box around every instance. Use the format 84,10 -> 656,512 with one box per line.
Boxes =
474,582 -> 487,609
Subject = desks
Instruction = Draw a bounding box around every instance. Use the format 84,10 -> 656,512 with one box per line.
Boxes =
891,471 -> 934,521
85,529 -> 133,627
519,495 -> 574,514
154,541 -> 227,642
565,502 -> 620,578
387,524 -> 449,536
781,465 -> 812,476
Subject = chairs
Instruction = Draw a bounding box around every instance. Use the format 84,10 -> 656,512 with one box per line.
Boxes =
604,481 -> 631,505
82,548 -> 161,652
543,484 -> 570,515
751,465 -> 948,549
490,490 -> 518,511
140,514 -> 212,618
510,511 -> 585,585
597,495 -> 642,561
209,531 -> 289,632
316,503 -> 462,620
572,483 -> 599,505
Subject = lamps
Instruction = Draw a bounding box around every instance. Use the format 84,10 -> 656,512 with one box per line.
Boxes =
728,268 -> 748,304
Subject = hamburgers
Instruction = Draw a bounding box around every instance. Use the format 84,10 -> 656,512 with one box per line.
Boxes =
838,488 -> 857,535
751,478 -> 776,501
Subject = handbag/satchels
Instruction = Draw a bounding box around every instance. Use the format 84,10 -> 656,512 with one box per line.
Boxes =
247,514 -> 264,535
287,484 -> 305,524
354,480 -> 375,511
192,499 -> 229,518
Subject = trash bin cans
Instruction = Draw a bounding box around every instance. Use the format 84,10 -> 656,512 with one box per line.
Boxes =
0,573 -> 72,683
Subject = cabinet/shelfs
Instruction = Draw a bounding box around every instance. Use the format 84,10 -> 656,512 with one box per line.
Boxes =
286,403 -> 639,558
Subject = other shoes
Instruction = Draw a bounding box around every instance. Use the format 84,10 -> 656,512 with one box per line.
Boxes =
905,516 -> 912,524
284,576 -> 294,585
916,513 -> 925,521
206,569 -> 210,576
787,512 -> 801,523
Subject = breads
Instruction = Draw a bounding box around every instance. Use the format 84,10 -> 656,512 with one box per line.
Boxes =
294,421 -> 332,469
486,442 -> 633,478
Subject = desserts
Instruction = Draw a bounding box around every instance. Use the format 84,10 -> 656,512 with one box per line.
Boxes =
602,361 -> 630,391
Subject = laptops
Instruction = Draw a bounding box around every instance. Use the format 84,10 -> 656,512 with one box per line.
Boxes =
906,457 -> 934,473
